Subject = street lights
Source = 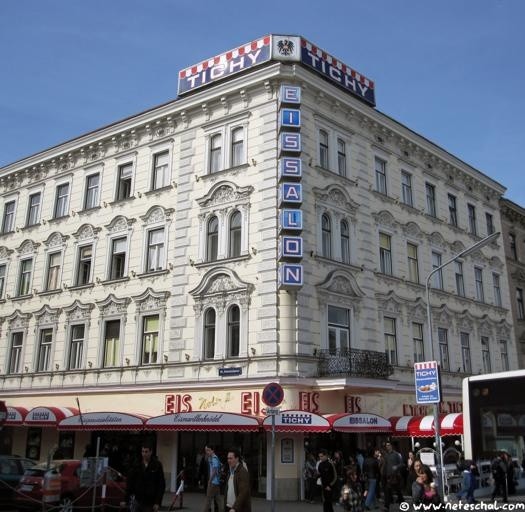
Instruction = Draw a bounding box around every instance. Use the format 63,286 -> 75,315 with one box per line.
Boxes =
423,229 -> 502,510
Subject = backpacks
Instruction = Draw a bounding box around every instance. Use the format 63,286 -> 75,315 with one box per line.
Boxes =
213,456 -> 227,484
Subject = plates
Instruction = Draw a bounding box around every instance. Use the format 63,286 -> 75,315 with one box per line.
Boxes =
417,386 -> 435,393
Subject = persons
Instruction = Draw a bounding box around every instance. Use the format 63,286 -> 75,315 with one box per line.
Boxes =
304,438 -> 519,511
202,443 -> 223,512
83,443 -> 136,483
223,450 -> 252,512
127,445 -> 165,512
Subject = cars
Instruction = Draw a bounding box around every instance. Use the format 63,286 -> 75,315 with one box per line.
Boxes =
441,449 -> 524,495
16,458 -> 127,512
0,454 -> 36,508
